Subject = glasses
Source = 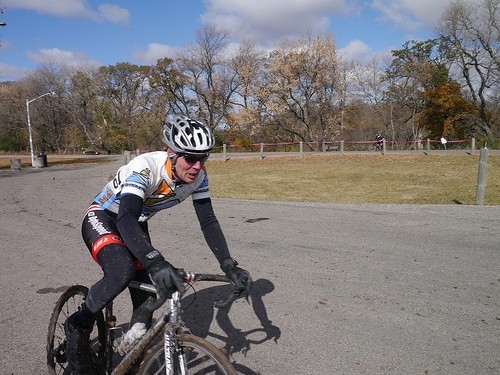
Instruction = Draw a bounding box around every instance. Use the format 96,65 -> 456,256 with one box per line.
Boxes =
181,155 -> 210,164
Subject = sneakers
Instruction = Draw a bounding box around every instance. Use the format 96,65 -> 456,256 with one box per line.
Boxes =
63,306 -> 96,372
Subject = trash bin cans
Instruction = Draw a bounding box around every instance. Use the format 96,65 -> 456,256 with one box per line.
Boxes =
37,152 -> 47,168
124,151 -> 131,165
135,148 -> 140,156
10,159 -> 21,170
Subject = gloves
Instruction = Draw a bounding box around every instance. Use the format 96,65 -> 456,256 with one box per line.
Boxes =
144,258 -> 187,299
220,257 -> 253,296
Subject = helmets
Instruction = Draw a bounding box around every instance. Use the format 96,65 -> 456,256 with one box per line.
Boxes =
162,114 -> 215,157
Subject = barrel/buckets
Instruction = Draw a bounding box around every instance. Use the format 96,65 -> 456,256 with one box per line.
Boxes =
10,158 -> 21,170
37,155 -> 47,167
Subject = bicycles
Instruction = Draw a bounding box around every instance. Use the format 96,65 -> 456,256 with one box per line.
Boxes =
45,267 -> 240,375
368,142 -> 383,152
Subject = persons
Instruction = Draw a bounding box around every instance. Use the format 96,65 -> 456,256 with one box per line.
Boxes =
64,114 -> 253,375
375,134 -> 382,148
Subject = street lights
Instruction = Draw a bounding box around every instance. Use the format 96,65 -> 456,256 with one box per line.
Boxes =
25,90 -> 56,168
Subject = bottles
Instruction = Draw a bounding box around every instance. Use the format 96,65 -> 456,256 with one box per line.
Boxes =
115,322 -> 147,357
